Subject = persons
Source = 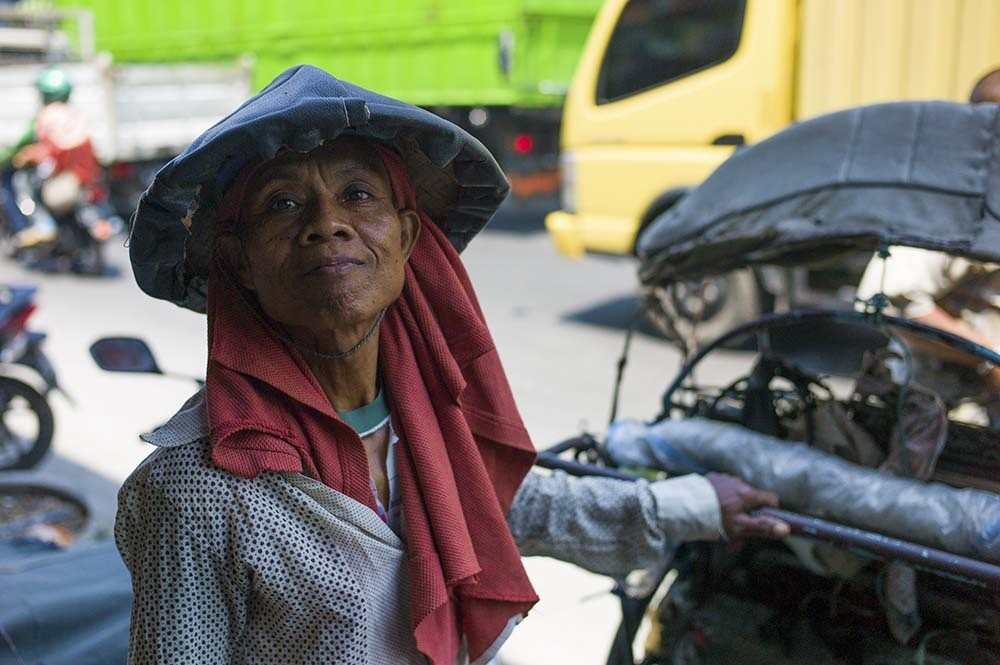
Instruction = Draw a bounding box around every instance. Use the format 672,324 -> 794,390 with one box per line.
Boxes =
10,70 -> 122,252
1,112 -> 45,259
848,66 -> 1000,429
111,63 -> 794,662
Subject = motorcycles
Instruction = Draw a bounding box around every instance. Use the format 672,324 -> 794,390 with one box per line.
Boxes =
0,283 -> 79,471
0,337 -> 207,665
0,144 -> 128,276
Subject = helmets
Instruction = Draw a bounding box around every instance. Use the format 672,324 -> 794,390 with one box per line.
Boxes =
33,67 -> 72,105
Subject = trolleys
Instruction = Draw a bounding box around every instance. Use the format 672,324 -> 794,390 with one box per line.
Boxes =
524,98 -> 998,665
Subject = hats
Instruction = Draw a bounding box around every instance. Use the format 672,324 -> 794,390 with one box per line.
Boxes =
123,64 -> 519,311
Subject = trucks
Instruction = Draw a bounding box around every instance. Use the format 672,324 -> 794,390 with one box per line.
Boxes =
16,0 -> 610,233
543,0 -> 1000,346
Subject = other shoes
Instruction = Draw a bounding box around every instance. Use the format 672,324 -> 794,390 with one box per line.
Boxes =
10,227 -> 57,247
